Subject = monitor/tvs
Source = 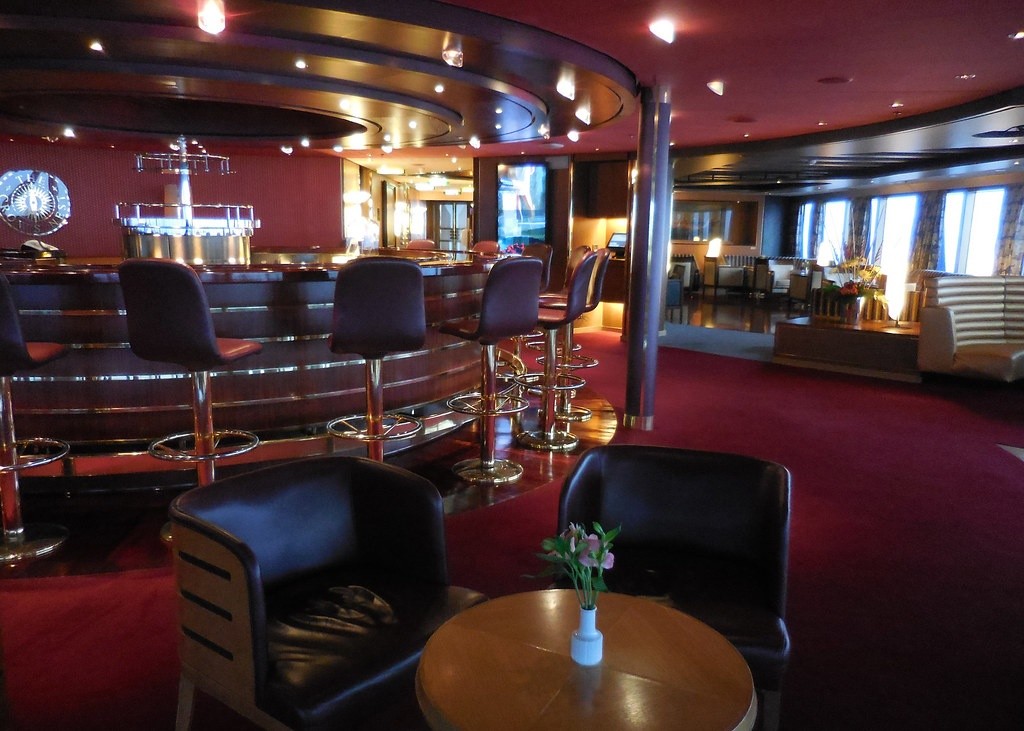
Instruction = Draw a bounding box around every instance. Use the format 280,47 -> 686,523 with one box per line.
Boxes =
606,233 -> 627,259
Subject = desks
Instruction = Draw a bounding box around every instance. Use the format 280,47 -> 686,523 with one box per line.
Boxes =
415,589 -> 758,731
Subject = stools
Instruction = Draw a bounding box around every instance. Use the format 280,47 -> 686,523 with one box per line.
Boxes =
535,248 -> 611,422
0,271 -> 70,568
526,245 -> 591,399
118,257 -> 263,544
495,245 -> 553,380
326,257 -> 425,463
438,255 -> 543,486
512,251 -> 598,450
473,241 -> 500,254
407,239 -> 436,251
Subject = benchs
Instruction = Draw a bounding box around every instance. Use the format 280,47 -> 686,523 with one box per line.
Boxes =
916,275 -> 1024,392
724,255 -> 807,270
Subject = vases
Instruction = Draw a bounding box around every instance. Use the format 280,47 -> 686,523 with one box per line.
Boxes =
569,603 -> 603,665
840,298 -> 861,324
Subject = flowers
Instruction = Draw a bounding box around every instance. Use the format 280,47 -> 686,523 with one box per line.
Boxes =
532,520 -> 623,610
820,205 -> 888,304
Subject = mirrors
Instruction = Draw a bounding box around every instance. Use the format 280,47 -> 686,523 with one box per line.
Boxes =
671,199 -> 759,247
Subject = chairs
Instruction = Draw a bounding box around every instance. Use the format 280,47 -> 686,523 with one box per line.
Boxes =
546,444 -> 791,731
702,257 -> 749,306
165,455 -> 490,731
666,279 -> 683,325
786,266 -> 823,319
750,259 -> 795,315
